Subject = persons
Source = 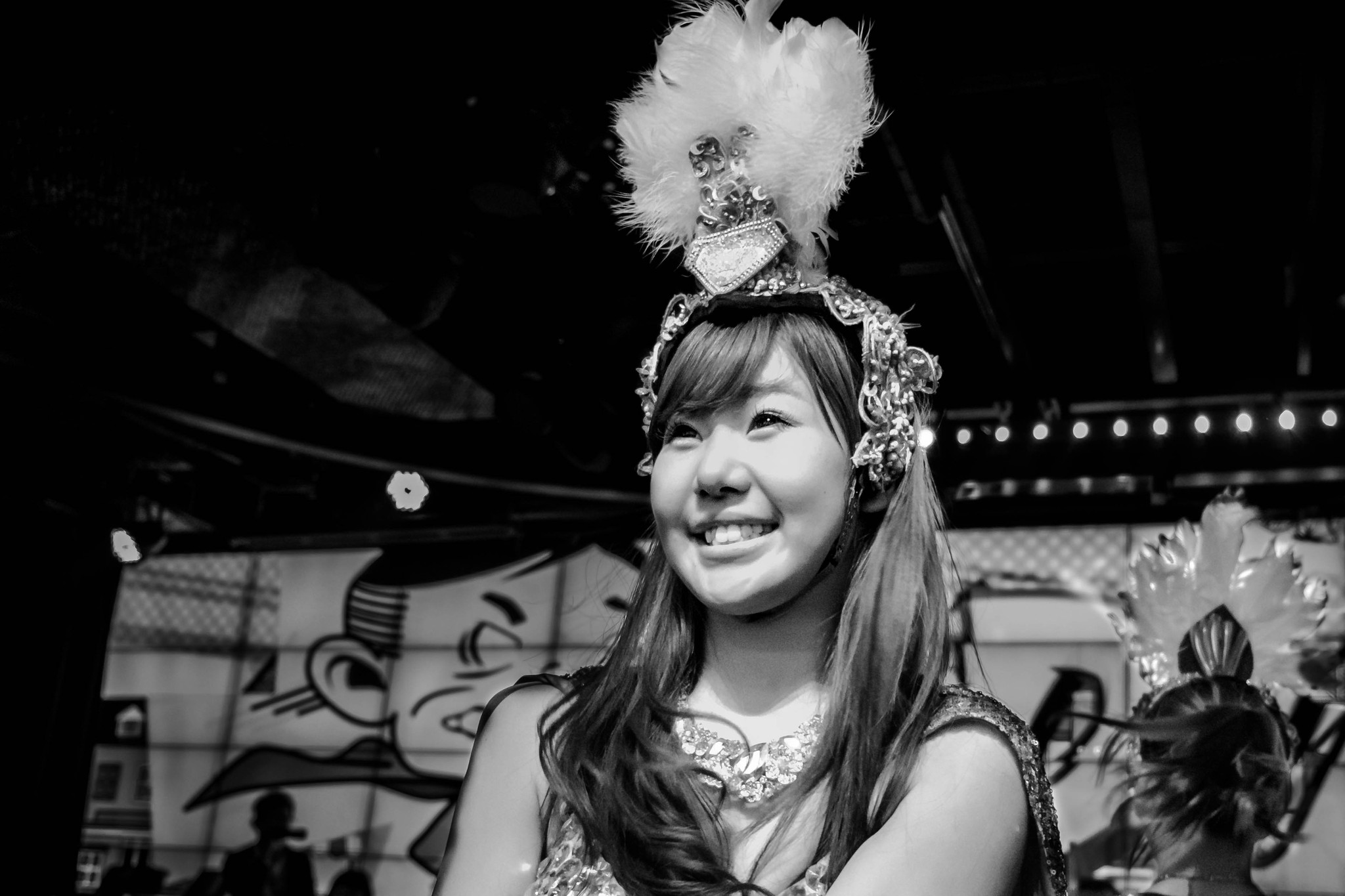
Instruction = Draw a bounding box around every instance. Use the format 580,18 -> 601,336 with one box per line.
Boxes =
1087,496 -> 1322,896
437,0 -> 1074,895
220,792 -> 315,896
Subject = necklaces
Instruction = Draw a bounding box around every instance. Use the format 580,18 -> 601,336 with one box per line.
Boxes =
672,684 -> 824,802
1153,871 -> 1252,885
